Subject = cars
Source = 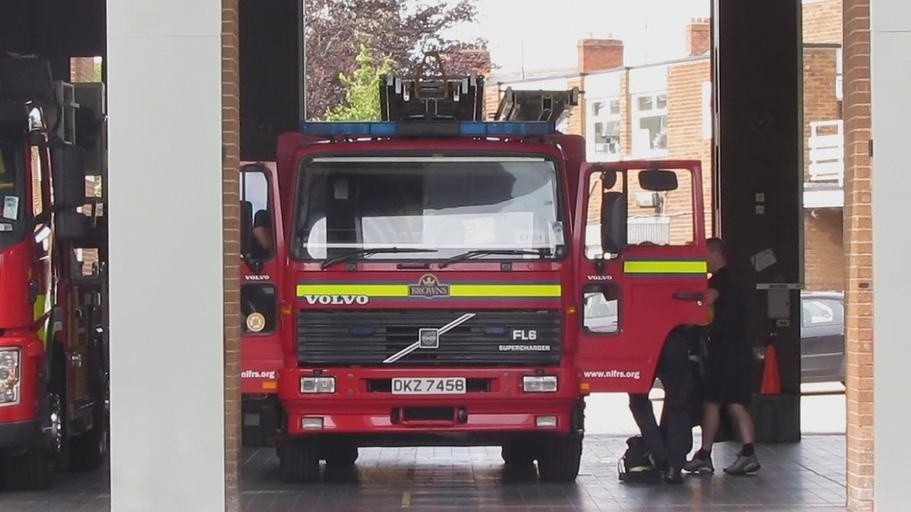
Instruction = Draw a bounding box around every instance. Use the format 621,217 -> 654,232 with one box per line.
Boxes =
792,286 -> 852,394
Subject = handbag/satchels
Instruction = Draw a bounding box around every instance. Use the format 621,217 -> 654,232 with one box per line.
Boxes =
618,434 -> 662,483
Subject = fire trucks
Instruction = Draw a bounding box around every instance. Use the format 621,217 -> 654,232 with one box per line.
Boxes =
0,46 -> 113,491
234,49 -> 714,486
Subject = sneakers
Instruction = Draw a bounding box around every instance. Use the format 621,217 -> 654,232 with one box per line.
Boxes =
682,456 -> 715,474
723,453 -> 761,475
651,459 -> 684,483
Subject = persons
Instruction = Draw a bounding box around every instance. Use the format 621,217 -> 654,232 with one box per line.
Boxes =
626,323 -> 703,485
681,233 -> 763,475
